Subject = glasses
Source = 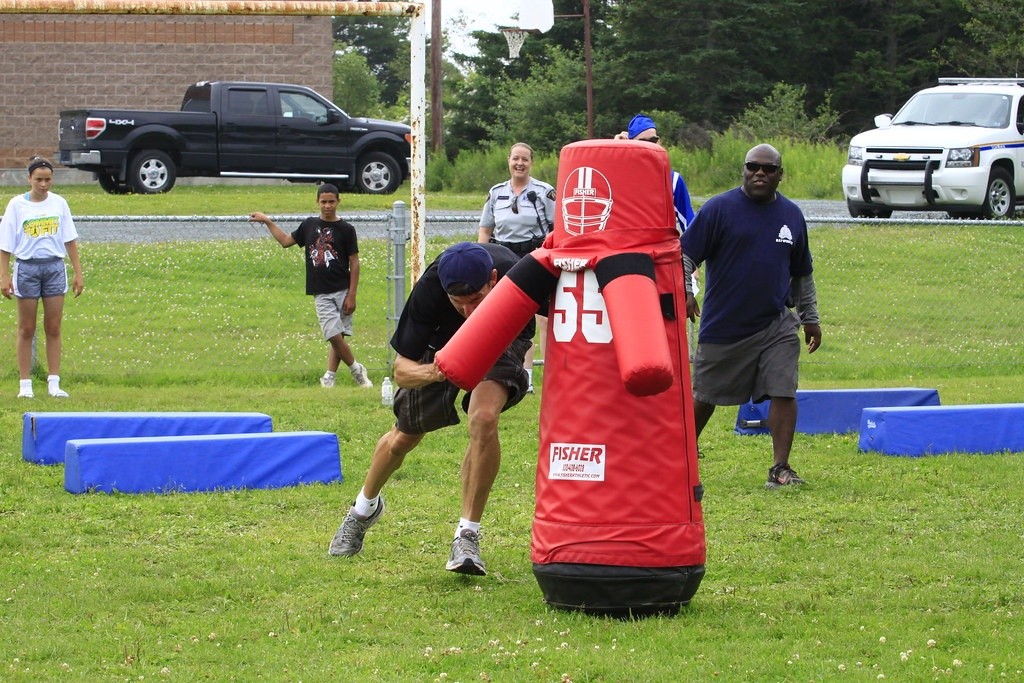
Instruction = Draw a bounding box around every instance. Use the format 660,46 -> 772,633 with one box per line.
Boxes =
511,195 -> 521,215
746,161 -> 783,175
637,135 -> 660,143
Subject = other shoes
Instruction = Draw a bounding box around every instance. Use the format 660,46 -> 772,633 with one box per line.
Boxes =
319,375 -> 333,388
352,363 -> 372,389
17,389 -> 36,399
49,387 -> 69,398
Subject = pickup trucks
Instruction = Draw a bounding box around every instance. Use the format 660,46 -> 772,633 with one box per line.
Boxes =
57,80 -> 411,194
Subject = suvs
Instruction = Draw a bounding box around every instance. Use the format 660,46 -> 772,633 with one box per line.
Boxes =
842,77 -> 1024,220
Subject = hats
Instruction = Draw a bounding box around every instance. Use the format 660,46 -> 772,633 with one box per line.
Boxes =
438,241 -> 493,296
627,114 -> 657,139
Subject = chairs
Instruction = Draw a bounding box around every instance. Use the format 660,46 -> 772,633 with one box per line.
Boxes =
258,95 -> 267,114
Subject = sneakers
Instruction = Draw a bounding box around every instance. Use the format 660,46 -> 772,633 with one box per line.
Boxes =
763,462 -> 807,489
444,527 -> 486,575
326,496 -> 386,557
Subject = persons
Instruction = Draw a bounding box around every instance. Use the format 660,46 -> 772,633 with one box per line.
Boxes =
614,113 -> 700,297
478,142 -> 557,393
327,242 -> 548,576
0,153 -> 83,397
679,144 -> 822,490
251,184 -> 373,387
